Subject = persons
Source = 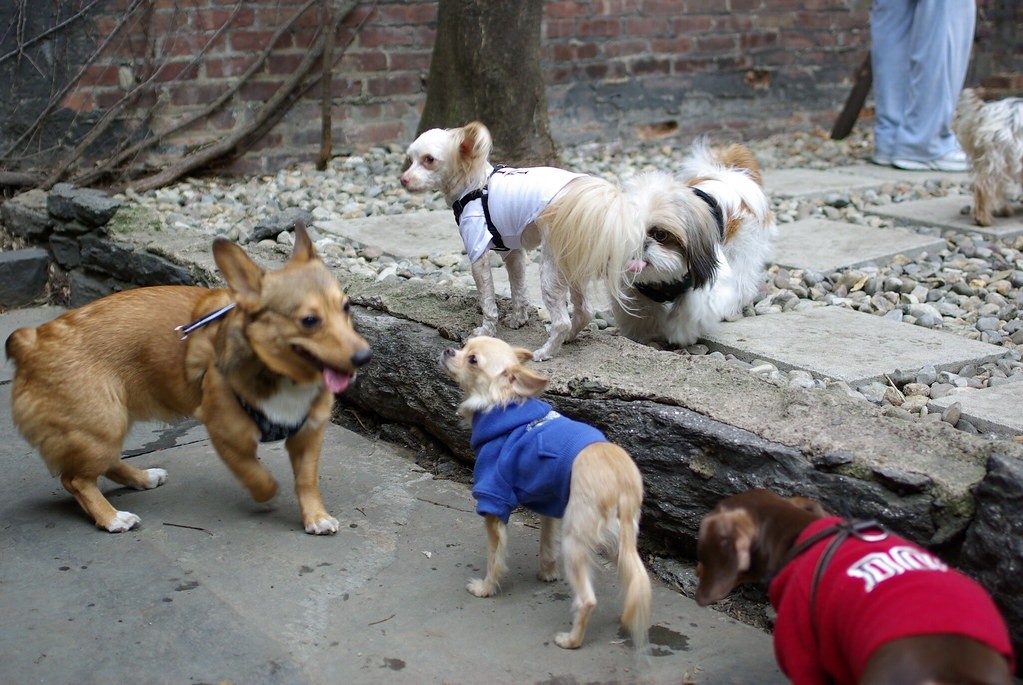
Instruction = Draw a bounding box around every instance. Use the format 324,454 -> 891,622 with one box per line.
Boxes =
865,0 -> 977,170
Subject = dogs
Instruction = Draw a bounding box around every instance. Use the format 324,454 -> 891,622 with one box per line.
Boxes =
399,121 -> 645,363
5,220 -> 374,536
694,492 -> 1017,685
611,140 -> 776,345
950,89 -> 1023,226
439,336 -> 653,651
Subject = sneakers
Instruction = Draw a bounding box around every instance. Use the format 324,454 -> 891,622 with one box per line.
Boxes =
893,148 -> 971,172
871,150 -> 894,165
